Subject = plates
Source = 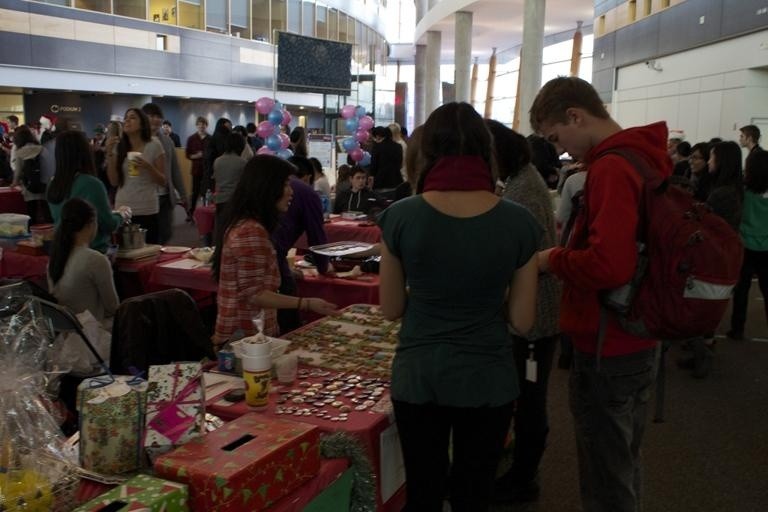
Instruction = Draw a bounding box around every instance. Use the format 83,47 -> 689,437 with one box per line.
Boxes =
161,246 -> 191,254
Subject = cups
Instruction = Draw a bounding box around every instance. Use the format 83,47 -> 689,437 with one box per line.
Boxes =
240,353 -> 273,411
127,152 -> 142,178
218,350 -> 235,372
287,247 -> 298,270
276,355 -> 298,383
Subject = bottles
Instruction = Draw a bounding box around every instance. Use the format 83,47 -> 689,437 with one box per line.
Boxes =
205,189 -> 213,207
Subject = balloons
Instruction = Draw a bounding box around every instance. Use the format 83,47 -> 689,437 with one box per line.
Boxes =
341,104 -> 372,171
255,98 -> 293,161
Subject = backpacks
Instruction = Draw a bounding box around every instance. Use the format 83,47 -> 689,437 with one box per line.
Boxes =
560,148 -> 745,340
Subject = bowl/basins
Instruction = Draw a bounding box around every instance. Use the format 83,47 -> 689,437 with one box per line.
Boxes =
113,224 -> 148,250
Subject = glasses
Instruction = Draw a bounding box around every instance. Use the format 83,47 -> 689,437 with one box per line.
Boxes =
689,155 -> 705,160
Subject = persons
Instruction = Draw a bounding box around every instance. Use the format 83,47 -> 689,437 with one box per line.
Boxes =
208,155 -> 340,355
484,118 -> 562,503
1,104 -> 266,255
529,77 -> 673,512
292,121 -> 426,216
486,118 -> 588,246
375,102 -> 549,512
45,196 -> 119,436
667,124 -> 768,341
